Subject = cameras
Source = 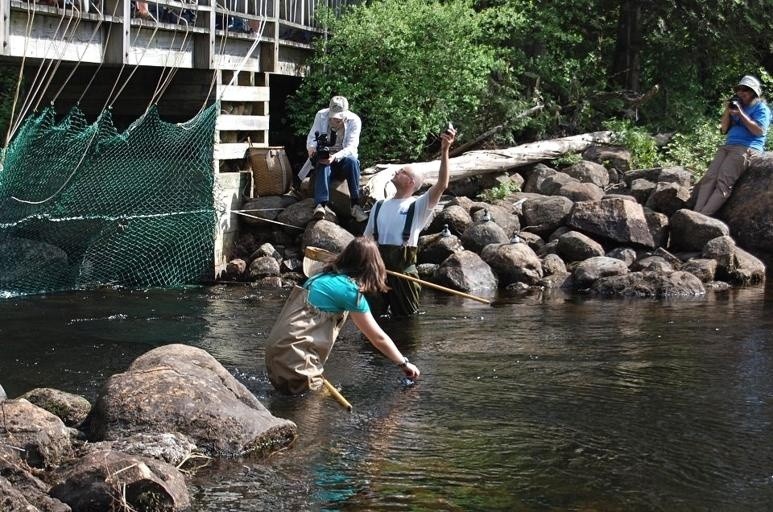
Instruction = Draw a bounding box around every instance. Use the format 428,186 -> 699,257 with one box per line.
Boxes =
729,95 -> 744,110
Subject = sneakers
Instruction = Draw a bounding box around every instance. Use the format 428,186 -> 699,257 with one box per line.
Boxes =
350,204 -> 368,222
313,204 -> 325,219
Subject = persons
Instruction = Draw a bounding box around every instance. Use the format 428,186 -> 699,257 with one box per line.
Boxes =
690,75 -> 770,216
305,95 -> 368,224
361,122 -> 457,318
262,233 -> 421,397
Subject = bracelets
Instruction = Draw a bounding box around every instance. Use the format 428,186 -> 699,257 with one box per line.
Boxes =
397,356 -> 408,368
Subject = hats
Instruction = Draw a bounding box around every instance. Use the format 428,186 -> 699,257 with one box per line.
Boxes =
737,75 -> 762,97
327,95 -> 349,121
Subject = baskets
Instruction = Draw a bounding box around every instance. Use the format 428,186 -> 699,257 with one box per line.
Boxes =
246,137 -> 294,196
